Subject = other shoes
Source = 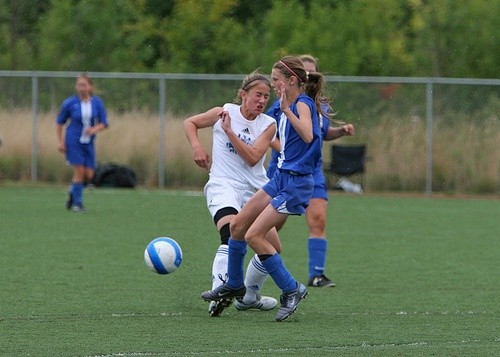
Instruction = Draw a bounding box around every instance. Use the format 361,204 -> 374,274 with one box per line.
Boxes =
70,206 -> 86,213
66,191 -> 72,208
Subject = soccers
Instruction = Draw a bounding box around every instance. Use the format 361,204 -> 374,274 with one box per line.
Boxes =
144,236 -> 183,274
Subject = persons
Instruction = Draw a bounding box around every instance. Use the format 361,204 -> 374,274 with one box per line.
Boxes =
55,75 -> 110,214
181,72 -> 278,317
199,54 -> 355,322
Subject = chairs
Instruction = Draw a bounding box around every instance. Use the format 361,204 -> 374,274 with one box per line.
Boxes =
324,144 -> 367,192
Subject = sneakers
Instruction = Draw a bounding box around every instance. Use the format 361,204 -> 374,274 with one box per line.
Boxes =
233,298 -> 279,311
209,299 -> 235,316
202,274 -> 246,298
275,282 -> 308,321
309,274 -> 336,287
